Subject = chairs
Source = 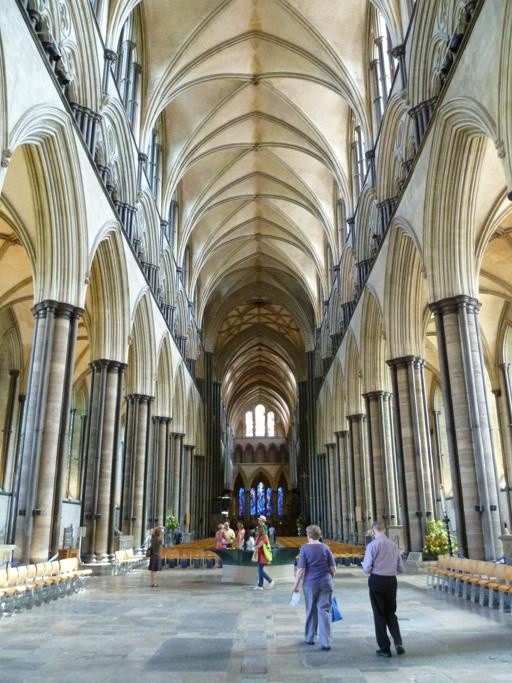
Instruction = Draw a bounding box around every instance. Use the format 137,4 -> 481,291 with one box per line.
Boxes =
0,557 -> 93,617
428,554 -> 512,615
115,548 -> 144,576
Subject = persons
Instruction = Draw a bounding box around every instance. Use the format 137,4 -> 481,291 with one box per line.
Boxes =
319,536 -> 323,543
361,521 -> 406,657
292,524 -> 336,651
214,515 -> 278,560
140,528 -> 183,569
253,526 -> 275,591
148,527 -> 163,587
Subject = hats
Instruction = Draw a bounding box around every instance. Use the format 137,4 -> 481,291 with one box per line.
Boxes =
257,515 -> 267,522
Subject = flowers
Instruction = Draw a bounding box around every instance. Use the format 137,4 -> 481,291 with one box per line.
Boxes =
422,518 -> 459,557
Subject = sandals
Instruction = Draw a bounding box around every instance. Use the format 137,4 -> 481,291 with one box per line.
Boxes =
149,582 -> 161,587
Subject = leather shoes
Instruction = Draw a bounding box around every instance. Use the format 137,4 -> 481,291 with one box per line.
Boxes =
376,645 -> 405,658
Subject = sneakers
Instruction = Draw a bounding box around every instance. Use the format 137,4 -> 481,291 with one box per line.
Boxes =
253,579 -> 275,590
304,638 -> 330,651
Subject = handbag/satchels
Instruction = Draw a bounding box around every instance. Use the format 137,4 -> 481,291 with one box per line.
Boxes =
251,543 -> 274,563
328,596 -> 343,623
146,547 -> 152,557
